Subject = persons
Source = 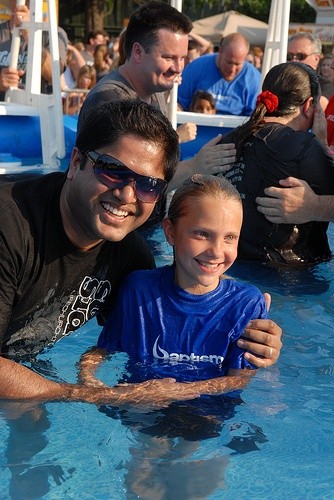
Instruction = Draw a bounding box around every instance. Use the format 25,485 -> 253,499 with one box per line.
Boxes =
0,98 -> 282,403
77,174 -> 270,411
0,0 -> 334,296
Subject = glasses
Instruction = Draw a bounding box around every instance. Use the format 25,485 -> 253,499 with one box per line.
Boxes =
86,149 -> 168,203
287,52 -> 316,61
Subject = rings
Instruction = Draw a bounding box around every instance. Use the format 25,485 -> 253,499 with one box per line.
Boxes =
266,347 -> 272,358
